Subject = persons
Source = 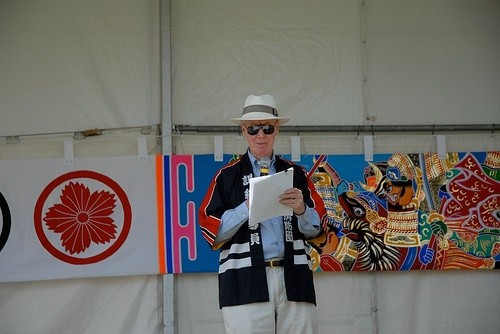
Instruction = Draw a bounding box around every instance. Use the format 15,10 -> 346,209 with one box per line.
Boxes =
196,94 -> 329,334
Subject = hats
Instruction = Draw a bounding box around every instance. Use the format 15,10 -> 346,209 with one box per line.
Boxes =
230,94 -> 290,125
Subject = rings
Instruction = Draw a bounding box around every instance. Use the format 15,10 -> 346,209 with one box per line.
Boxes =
292,199 -> 295,204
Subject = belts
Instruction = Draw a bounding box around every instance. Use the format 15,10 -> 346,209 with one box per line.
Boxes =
263,257 -> 285,267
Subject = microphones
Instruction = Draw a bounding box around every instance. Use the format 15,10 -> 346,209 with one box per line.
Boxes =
259,156 -> 271,176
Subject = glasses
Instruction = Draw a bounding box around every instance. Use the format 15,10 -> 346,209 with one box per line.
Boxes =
243,122 -> 278,134
